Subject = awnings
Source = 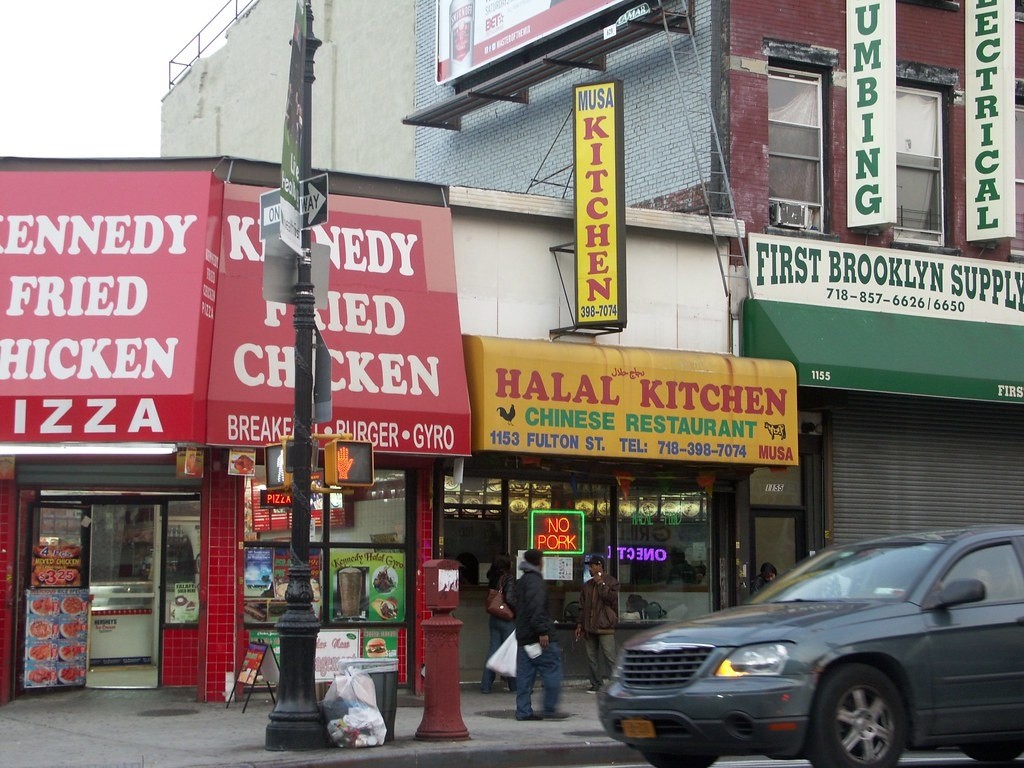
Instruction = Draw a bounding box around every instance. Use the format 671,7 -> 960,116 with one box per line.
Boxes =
463,335 -> 799,468
741,298 -> 1024,405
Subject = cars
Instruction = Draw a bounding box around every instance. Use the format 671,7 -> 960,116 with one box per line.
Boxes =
598,525 -> 1024,768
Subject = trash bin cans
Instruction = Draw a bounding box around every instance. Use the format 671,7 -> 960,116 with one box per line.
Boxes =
336,658 -> 400,742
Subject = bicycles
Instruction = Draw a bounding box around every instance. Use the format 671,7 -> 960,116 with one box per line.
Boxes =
565,601 -> 581,623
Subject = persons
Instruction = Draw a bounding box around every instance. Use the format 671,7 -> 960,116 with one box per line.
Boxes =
752,561 -> 781,597
481,552 -> 517,694
663,547 -> 697,585
575,555 -> 618,693
514,549 -> 571,721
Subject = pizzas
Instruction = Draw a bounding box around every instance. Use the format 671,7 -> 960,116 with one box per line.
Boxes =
29,597 -> 84,685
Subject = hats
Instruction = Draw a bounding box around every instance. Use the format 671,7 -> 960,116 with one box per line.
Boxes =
582,556 -> 605,567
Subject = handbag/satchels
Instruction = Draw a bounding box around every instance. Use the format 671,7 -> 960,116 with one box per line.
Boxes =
485,630 -> 518,678
486,573 -> 514,620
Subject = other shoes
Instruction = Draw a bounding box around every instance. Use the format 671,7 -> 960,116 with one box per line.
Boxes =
517,713 -> 543,720
587,687 -> 596,694
542,709 -> 570,719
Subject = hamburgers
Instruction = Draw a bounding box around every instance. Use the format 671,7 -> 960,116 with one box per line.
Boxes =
365,638 -> 388,657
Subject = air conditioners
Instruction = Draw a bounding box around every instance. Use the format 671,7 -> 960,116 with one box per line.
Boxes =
770,200 -> 808,229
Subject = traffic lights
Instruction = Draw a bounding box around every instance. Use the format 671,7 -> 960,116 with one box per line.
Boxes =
326,437 -> 375,489
263,442 -> 289,492
258,171 -> 331,244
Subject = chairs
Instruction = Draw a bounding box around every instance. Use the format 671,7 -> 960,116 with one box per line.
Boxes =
646,601 -> 664,619
563,601 -> 581,624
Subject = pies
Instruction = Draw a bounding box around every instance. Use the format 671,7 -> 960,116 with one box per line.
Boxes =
371,598 -> 399,619
